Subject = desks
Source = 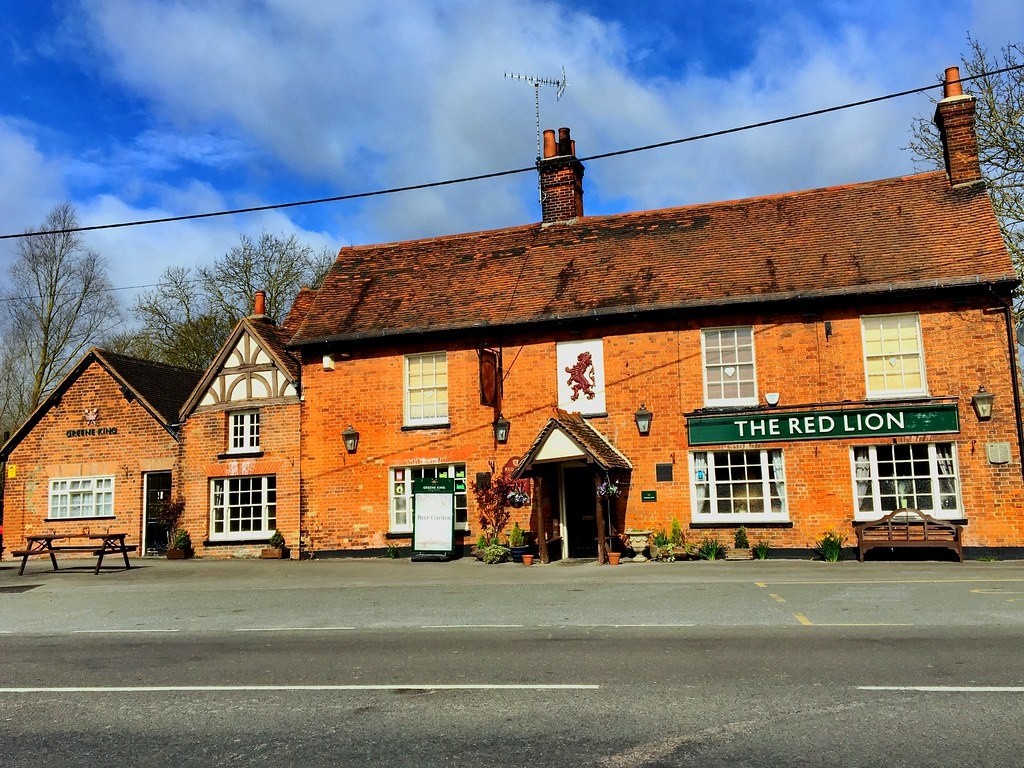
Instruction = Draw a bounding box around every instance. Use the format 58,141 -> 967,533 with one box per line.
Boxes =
17,533 -> 131,575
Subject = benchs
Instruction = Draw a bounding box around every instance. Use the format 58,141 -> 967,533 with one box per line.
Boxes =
11,550 -> 102,557
855,508 -> 964,564
45,545 -> 138,552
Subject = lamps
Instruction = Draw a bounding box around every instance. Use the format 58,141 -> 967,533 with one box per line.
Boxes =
341,424 -> 359,453
632,403 -> 653,435
491,413 -> 510,444
970,385 -> 995,422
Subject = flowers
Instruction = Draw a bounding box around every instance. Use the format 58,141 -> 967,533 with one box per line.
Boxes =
596,482 -> 622,498
507,483 -> 531,504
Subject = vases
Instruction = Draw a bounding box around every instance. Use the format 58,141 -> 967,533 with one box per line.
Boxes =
607,552 -> 621,565
522,555 -> 535,565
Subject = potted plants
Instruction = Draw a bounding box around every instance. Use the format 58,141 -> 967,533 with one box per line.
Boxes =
725,525 -> 754,561
509,521 -> 529,563
624,529 -> 653,562
166,528 -> 194,559
261,529 -> 287,559
